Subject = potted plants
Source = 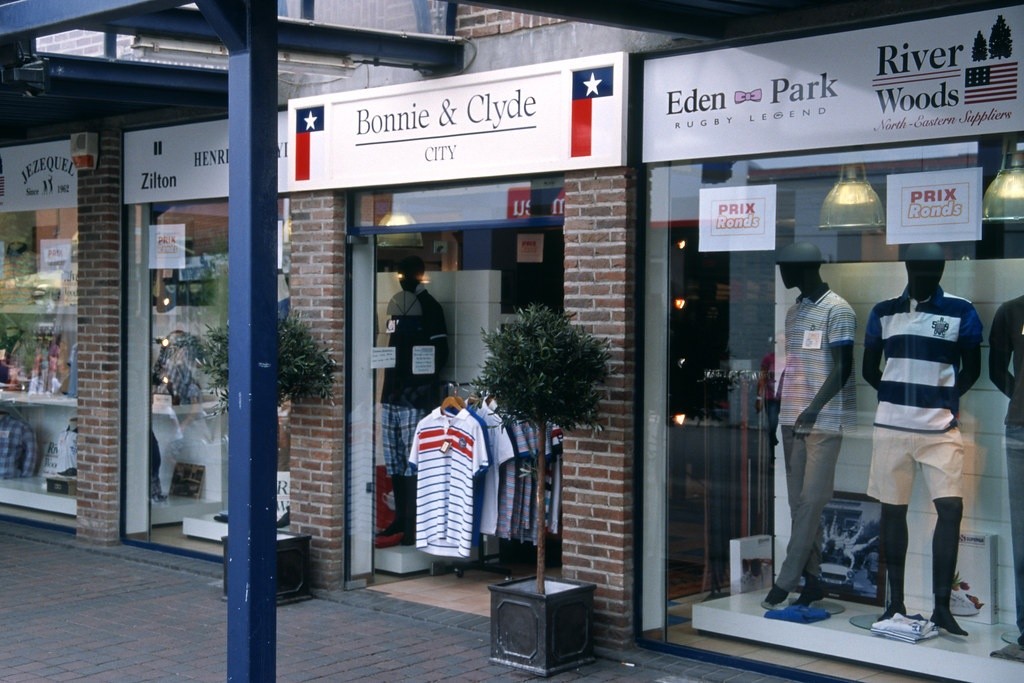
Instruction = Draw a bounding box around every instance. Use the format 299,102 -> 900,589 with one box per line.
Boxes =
476,303 -> 616,676
194,313 -> 340,607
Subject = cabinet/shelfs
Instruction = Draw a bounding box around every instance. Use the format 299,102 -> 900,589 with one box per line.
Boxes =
147,303 -> 228,542
0,302 -> 79,519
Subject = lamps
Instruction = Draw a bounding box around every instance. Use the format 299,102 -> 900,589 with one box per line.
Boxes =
817,164 -> 885,232
983,133 -> 1023,222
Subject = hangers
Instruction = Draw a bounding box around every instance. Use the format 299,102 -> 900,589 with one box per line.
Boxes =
440,382 -> 466,413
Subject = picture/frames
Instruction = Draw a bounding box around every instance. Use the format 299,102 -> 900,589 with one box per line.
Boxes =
816,491 -> 888,607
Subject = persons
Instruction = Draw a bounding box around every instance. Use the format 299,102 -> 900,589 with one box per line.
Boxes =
765,240 -> 858,607
862,243 -> 982,636
989,295 -> 1024,645
376,255 -> 450,546
